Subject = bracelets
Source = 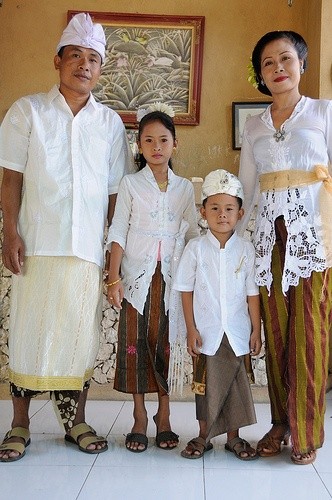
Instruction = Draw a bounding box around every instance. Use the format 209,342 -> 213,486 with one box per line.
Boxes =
106,278 -> 121,287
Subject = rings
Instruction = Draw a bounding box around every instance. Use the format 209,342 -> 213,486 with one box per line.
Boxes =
110,297 -> 113,299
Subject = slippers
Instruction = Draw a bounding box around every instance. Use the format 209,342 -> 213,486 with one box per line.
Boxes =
65,423 -> 108,454
181,437 -> 213,459
125,433 -> 148,453
0,426 -> 31,462
153,415 -> 180,451
225,437 -> 258,460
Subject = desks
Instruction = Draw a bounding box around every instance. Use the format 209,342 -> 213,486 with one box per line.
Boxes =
0,209 -> 272,404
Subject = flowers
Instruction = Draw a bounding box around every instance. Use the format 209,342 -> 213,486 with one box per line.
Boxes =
247,47 -> 259,89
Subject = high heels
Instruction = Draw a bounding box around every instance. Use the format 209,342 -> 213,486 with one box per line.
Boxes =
257,427 -> 291,456
291,445 -> 317,464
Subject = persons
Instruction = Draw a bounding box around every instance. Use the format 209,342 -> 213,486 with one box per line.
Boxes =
106,111 -> 202,453
235,30 -> 332,465
173,168 -> 262,461
0,12 -> 130,462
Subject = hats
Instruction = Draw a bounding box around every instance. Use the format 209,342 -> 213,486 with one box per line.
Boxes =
200,169 -> 244,207
56,12 -> 106,68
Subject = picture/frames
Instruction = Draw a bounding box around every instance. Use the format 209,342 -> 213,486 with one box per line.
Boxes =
67,10 -> 205,127
232,101 -> 273,151
